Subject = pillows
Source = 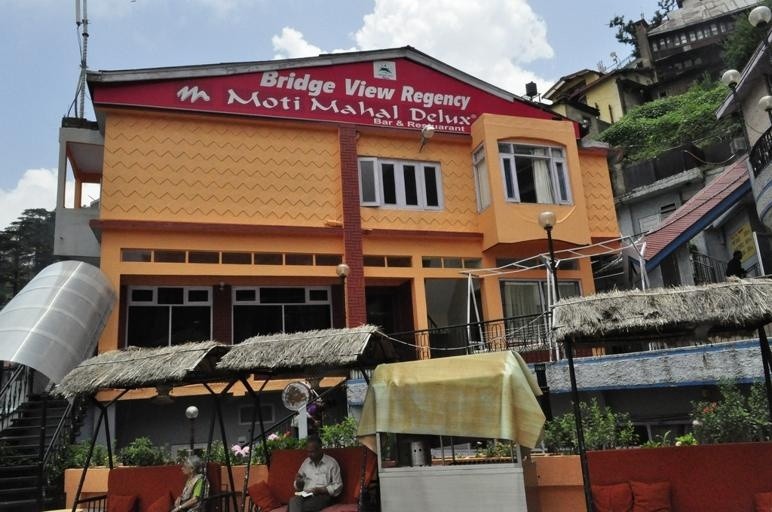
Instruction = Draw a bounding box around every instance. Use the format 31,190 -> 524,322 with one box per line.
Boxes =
589,481 -> 636,512
629,480 -> 673,511
107,493 -> 137,511
144,490 -> 174,512
246,478 -> 284,511
752,488 -> 772,511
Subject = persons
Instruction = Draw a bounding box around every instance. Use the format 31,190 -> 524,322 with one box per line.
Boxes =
288,435 -> 345,511
725,249 -> 747,278
170,456 -> 211,511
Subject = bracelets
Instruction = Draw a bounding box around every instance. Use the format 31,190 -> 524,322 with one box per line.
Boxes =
178,504 -> 182,509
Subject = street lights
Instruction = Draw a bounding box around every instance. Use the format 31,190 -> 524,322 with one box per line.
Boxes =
539,210 -> 573,359
759,95 -> 772,125
749,5 -> 772,69
722,69 -> 757,172
336,263 -> 351,378
185,405 -> 199,454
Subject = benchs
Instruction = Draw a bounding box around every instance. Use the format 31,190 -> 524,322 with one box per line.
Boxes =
65,458 -> 234,512
241,444 -> 380,512
584,440 -> 772,511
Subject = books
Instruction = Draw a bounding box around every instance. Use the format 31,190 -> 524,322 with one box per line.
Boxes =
295,489 -> 314,498
